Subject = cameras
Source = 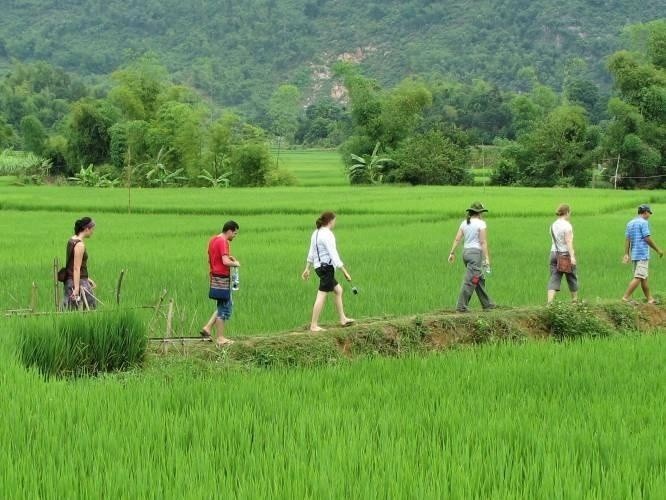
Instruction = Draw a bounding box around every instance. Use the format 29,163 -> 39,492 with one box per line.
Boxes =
351,286 -> 358,295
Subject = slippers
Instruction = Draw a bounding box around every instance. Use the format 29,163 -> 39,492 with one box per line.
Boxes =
200,329 -> 213,341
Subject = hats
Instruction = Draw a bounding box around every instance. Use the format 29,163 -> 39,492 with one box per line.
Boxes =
638,205 -> 652,214
466,202 -> 488,212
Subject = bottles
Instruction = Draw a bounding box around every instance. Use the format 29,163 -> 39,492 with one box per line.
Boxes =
481,256 -> 491,273
231,265 -> 239,291
346,279 -> 360,297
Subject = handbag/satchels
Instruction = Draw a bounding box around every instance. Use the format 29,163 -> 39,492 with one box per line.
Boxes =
209,275 -> 229,299
321,263 -> 327,273
557,256 -> 572,273
58,268 -> 67,281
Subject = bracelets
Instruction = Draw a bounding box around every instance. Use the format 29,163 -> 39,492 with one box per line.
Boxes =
450,252 -> 455,255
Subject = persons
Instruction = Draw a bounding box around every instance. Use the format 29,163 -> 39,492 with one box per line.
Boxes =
301,209 -> 356,333
621,203 -> 663,304
545,204 -> 581,307
60,216 -> 98,315
448,202 -> 500,315
199,221 -> 241,348
